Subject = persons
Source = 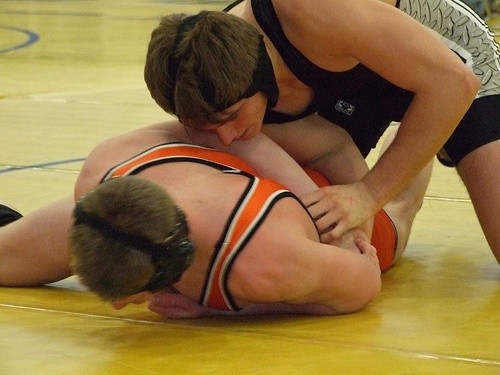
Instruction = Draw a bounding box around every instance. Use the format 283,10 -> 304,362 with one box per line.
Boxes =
0,120 -> 434,319
144,0 -> 500,263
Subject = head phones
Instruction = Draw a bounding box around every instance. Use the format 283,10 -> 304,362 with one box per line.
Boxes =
75,209 -> 195,283
